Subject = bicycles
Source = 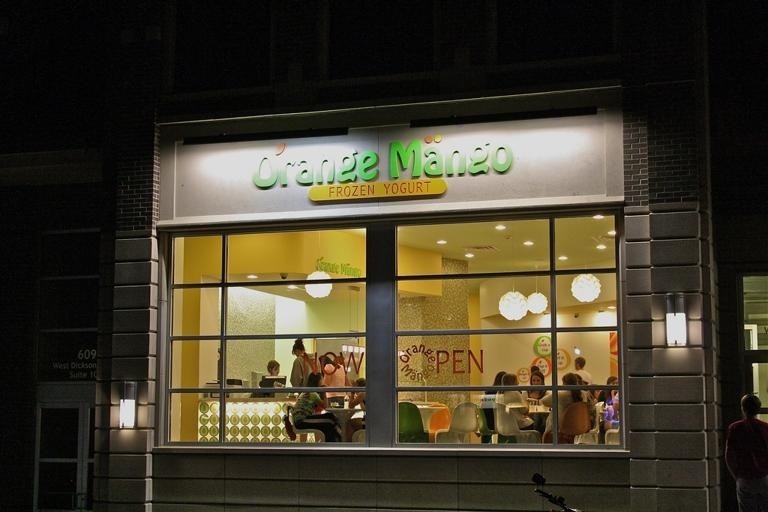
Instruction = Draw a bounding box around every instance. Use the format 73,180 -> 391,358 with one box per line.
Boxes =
531,472 -> 583,512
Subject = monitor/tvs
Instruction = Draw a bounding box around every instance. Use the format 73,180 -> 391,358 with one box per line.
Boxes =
261,374 -> 287,388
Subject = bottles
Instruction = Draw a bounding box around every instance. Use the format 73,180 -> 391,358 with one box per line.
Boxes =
478,394 -> 496,408
343,394 -> 350,408
526,397 -> 545,412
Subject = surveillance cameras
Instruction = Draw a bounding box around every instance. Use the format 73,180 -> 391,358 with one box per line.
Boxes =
280,274 -> 288,279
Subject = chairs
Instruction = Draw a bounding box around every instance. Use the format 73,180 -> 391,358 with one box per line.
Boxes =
398,399 -> 621,445
284,392 -> 366,442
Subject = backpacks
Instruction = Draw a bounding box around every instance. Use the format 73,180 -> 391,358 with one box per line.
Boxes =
283,405 -> 298,441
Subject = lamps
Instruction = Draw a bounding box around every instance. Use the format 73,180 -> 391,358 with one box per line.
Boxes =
524,264 -> 549,313
570,218 -> 602,302
341,283 -> 366,353
113,379 -> 140,432
304,230 -> 333,299
664,290 -> 688,347
499,235 -> 529,322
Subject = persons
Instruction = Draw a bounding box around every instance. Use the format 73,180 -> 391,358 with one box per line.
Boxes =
319,355 -> 346,408
292,372 -> 342,442
345,377 -> 367,444
482,357 -> 619,445
290,338 -> 322,443
267,359 -> 279,376
725,395 -> 768,512
